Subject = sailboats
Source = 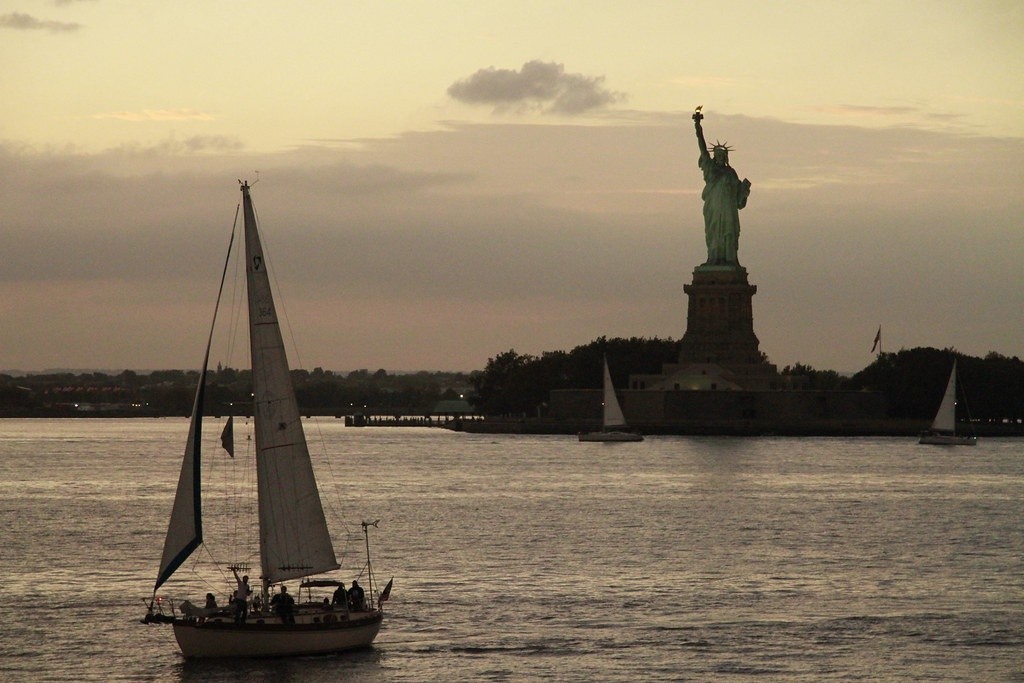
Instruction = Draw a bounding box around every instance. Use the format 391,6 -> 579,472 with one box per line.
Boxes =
137,171 -> 397,658
579,351 -> 646,442
917,359 -> 979,447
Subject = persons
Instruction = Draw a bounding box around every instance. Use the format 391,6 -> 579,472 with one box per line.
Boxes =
348,580 -> 364,612
277,586 -> 296,625
198,593 -> 217,623
693,113 -> 749,265
229,569 -> 253,627
331,585 -> 348,607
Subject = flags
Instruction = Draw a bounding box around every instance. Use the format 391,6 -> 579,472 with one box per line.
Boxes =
871,329 -> 880,352
